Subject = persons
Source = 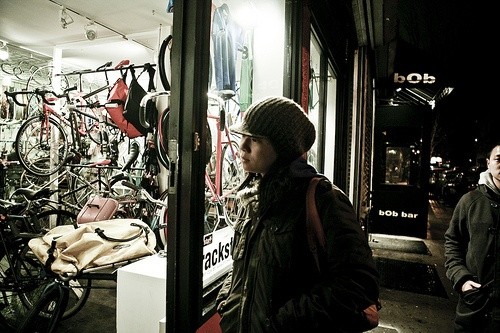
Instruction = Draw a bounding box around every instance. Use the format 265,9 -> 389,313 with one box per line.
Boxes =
214,97 -> 378,333
443,145 -> 500,333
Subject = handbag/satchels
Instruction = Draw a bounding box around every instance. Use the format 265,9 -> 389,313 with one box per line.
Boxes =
453,279 -> 499,325
308,176 -> 379,333
105,68 -> 155,138
28,194 -> 156,279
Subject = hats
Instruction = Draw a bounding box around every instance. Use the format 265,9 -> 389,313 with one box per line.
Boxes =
230,97 -> 316,157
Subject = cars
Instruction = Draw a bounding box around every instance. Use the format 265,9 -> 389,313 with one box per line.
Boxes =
431,164 -> 476,201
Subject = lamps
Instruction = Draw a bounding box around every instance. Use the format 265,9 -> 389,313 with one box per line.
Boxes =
84,17 -> 98,40
59,5 -> 74,29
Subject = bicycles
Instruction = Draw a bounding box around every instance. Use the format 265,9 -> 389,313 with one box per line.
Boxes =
0,60 -> 248,325
